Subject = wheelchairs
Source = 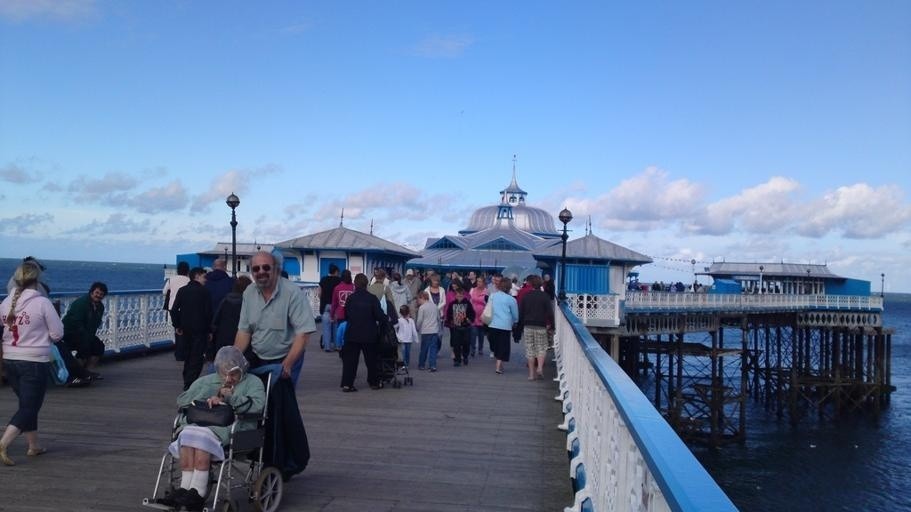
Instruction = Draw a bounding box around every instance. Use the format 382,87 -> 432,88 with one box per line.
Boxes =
140,370 -> 283,512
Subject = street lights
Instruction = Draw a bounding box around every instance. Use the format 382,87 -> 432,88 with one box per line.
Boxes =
758,265 -> 764,295
557,204 -> 573,301
225,190 -> 241,280
806,268 -> 810,294
879,271 -> 883,298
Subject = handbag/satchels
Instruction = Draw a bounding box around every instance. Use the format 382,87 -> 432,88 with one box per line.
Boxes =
186,400 -> 235,427
480,301 -> 493,324
46,344 -> 69,388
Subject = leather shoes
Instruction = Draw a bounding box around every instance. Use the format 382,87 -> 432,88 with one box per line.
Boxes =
496,370 -> 505,374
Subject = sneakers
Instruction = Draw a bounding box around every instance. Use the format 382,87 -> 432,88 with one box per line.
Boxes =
157,488 -> 202,512
27,447 -> 46,455
343,386 -> 358,391
0,441 -> 15,465
371,382 -> 383,389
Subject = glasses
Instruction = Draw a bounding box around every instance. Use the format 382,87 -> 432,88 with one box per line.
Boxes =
253,264 -> 270,272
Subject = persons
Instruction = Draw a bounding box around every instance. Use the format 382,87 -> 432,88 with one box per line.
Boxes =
0,255 -> 111,466
319,265 -> 554,392
628,279 -> 704,293
161,257 -> 251,391
232,251 -> 316,502
156,346 -> 264,512
